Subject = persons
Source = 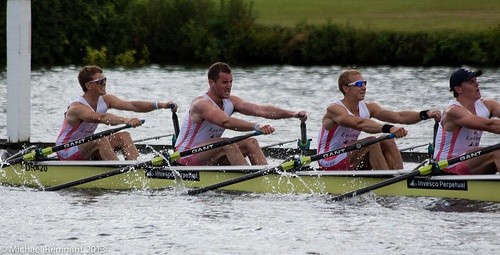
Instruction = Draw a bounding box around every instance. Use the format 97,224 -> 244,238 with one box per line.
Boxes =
318,70 -> 442,170
55,65 -> 179,160
434,69 -> 500,175
175,62 -> 308,165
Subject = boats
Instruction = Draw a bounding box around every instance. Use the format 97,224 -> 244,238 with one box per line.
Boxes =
0,139 -> 500,202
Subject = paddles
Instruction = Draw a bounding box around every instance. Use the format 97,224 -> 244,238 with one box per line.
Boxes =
298,114 -> 312,150
168,105 -> 180,137
0,117 -> 147,170
332,143 -> 500,202
36,127 -> 273,192
188,133 -> 397,197
432,117 -> 441,146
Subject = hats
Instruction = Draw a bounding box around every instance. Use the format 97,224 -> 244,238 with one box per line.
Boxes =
449,69 -> 483,92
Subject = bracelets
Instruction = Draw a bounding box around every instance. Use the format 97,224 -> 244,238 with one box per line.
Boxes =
154,102 -> 158,110
420,110 -> 430,120
254,124 -> 259,130
382,124 -> 394,133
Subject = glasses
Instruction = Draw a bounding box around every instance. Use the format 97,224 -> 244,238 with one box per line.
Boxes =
89,77 -> 106,84
347,80 -> 367,87
